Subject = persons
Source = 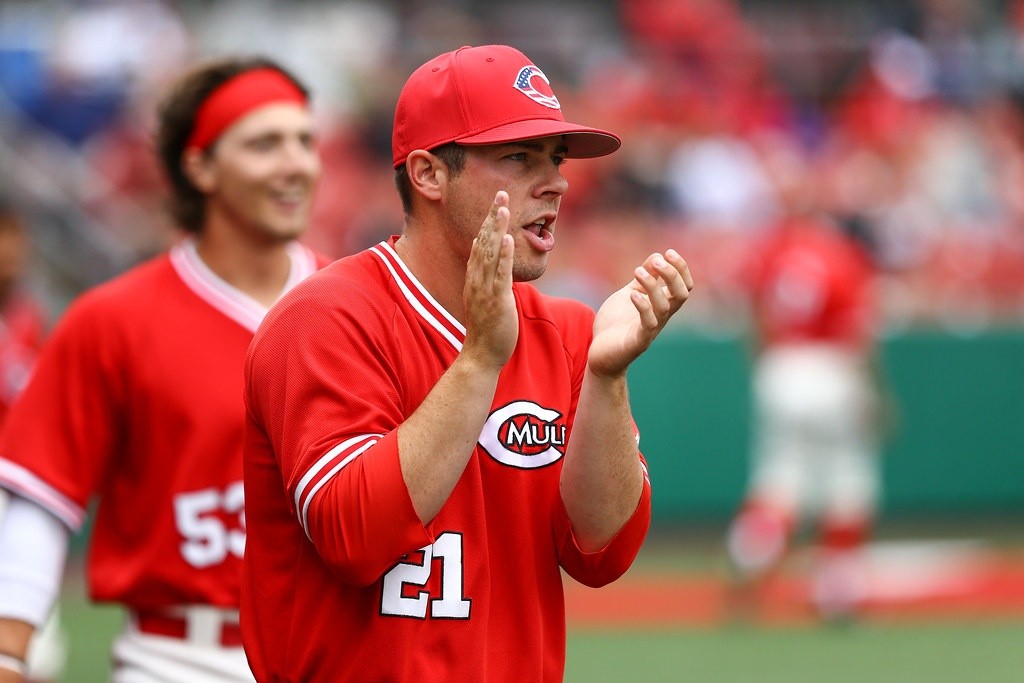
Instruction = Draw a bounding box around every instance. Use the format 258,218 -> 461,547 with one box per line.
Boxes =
242,45 -> 695,682
1,0 -> 1024,683
0,58 -> 330,683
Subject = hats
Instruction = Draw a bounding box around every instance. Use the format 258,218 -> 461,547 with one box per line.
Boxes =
392,45 -> 622,167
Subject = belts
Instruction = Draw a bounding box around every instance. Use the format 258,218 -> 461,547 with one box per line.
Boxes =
134,611 -> 245,649
